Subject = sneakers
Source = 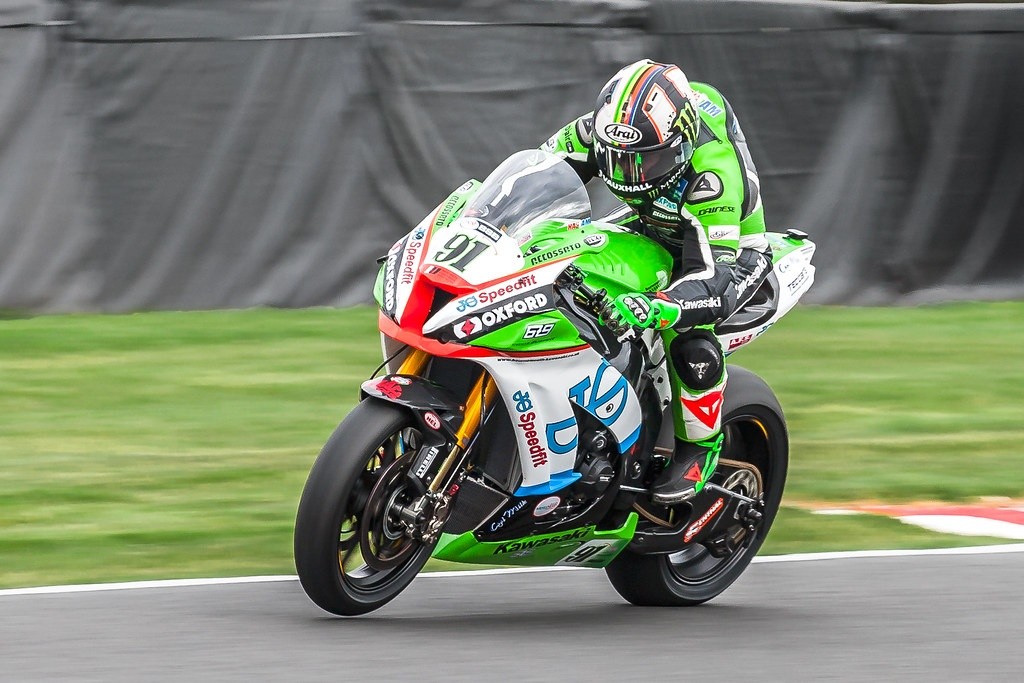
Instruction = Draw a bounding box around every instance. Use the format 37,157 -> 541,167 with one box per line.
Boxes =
652,433 -> 723,506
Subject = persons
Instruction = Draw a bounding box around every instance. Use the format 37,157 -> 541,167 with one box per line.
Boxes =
466,58 -> 770,503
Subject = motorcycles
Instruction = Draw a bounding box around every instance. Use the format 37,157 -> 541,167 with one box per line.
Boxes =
288,151 -> 818,617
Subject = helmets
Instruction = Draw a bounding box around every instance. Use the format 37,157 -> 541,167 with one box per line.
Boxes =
593,58 -> 699,202
